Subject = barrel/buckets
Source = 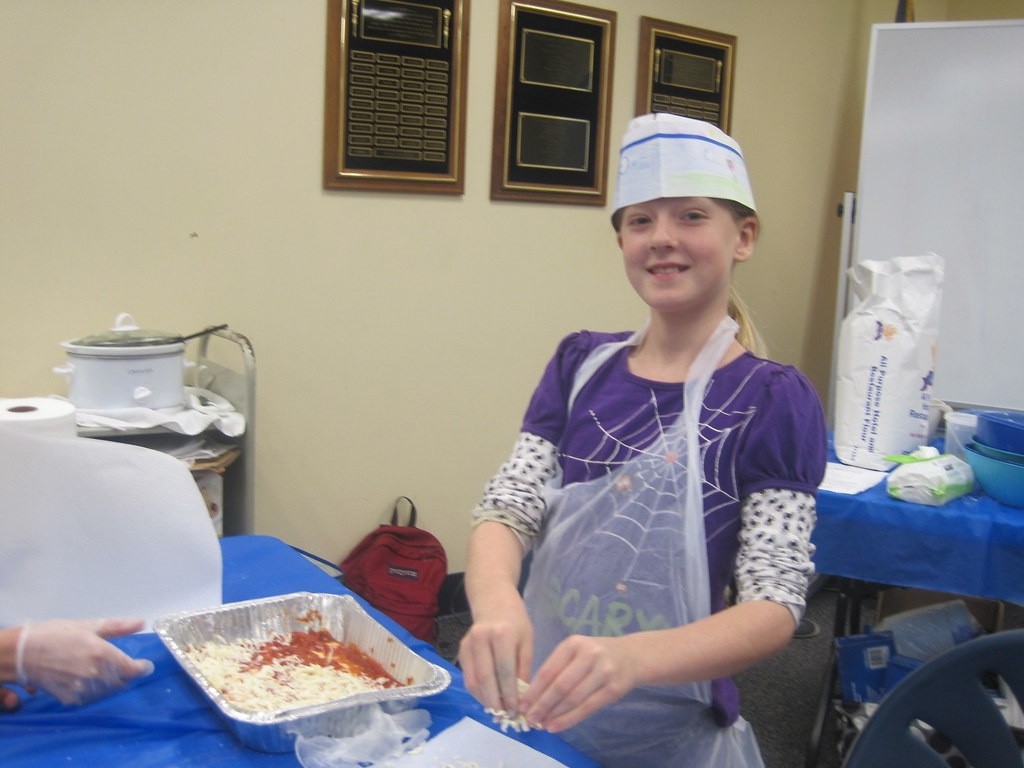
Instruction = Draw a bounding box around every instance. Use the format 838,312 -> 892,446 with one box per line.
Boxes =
942,411 -> 978,461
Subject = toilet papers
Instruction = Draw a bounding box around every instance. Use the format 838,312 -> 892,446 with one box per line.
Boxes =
0,396 -> 223,634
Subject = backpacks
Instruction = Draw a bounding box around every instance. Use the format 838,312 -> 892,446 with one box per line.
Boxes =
341,495 -> 449,643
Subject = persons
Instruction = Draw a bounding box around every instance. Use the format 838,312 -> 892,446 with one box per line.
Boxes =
460,112 -> 828,768
0,617 -> 150,710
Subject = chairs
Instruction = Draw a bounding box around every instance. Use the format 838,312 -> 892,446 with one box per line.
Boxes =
845,628 -> 1024,768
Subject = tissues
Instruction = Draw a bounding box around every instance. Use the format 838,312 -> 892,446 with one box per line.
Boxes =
879,445 -> 975,506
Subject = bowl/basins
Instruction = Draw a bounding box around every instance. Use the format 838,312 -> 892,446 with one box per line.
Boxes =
960,411 -> 1024,511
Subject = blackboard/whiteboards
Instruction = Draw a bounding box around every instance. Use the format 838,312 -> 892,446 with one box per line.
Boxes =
846,18 -> 1024,418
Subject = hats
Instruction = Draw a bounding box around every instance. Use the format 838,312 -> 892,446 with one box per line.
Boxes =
609,112 -> 760,239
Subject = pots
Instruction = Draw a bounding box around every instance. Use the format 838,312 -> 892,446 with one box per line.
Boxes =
52,315 -> 195,416
